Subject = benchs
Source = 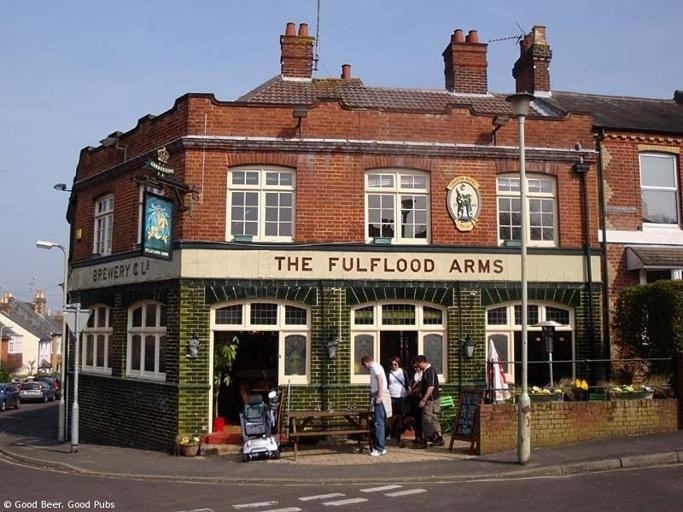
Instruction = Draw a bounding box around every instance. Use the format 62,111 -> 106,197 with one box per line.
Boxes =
287,409 -> 373,461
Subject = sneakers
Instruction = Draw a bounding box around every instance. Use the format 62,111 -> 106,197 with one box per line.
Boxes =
411,435 -> 445,449
369,446 -> 386,457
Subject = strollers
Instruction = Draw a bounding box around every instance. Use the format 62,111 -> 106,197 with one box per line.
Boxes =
239,401 -> 280,462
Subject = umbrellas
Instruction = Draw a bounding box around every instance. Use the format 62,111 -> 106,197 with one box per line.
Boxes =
487,341 -> 512,404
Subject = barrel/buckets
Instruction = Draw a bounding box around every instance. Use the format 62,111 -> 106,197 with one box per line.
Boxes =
213,416 -> 224,433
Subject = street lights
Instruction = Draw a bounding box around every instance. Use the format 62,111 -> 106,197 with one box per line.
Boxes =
506,92 -> 534,465
36,241 -> 68,441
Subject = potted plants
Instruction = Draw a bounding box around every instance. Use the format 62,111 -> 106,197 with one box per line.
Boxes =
175,431 -> 201,457
212,334 -> 239,432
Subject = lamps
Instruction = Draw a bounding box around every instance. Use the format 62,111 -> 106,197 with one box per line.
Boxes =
463,334 -> 475,358
490,114 -> 508,146
52,184 -> 72,193
292,110 -> 308,139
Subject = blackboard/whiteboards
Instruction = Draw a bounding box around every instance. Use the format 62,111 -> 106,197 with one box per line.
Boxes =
452,389 -> 484,440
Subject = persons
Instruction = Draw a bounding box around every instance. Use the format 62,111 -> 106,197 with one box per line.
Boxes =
417,355 -> 445,446
362,356 -> 393,456
386,355 -> 411,441
407,355 -> 426,444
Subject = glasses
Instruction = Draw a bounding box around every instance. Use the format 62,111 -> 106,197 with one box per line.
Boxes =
390,363 -> 397,367
412,366 -> 418,369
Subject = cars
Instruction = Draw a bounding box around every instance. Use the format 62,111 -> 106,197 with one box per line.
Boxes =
0,375 -> 61,411
